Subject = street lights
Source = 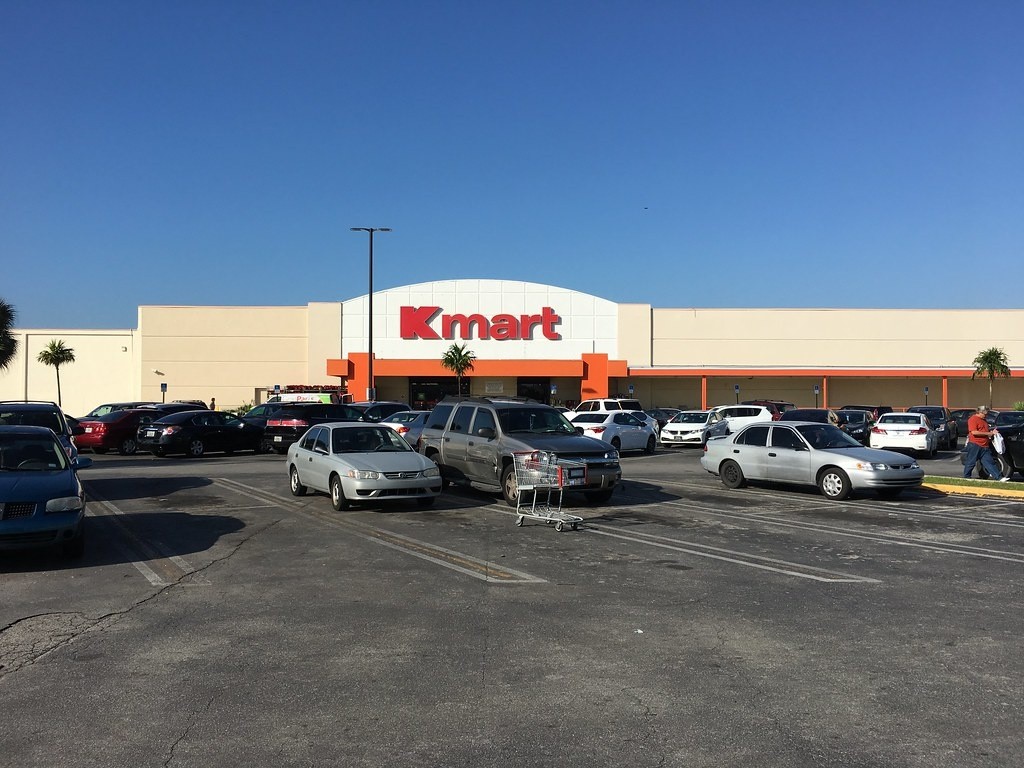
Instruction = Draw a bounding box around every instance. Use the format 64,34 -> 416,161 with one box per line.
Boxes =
350,226 -> 392,400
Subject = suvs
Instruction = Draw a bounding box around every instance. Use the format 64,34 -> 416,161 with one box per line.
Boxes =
344,401 -> 413,424
262,402 -> 381,454
419,394 -> 623,507
561,397 -> 644,422
740,399 -> 798,421
960,421 -> 1024,481
0,401 -> 78,465
777,404 -> 894,442
75,402 -> 211,423
907,405 -> 960,452
702,405 -> 774,433
227,399 -> 319,429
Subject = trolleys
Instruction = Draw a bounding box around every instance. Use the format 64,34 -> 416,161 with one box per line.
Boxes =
510,450 -> 584,533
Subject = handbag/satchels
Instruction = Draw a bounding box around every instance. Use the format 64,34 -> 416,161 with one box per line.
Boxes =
992,432 -> 1006,454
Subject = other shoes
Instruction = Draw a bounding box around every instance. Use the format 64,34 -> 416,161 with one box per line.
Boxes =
1000,477 -> 1010,482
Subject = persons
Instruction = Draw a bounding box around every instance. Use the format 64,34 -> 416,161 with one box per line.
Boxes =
210,398 -> 215,410
964,405 -> 1010,483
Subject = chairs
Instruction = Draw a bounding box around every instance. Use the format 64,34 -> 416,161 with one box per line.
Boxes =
886,419 -> 917,424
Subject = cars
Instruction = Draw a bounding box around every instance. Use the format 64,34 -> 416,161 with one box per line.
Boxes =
563,411 -> 657,454
285,422 -> 443,511
869,412 -> 940,460
553,396 -> 689,433
76,407 -> 169,456
404,413 -> 432,451
138,408 -> 271,459
63,414 -> 79,428
950,409 -> 1024,437
659,410 -> 731,449
376,411 -> 434,453
700,421 -> 925,501
0,423 -> 94,559
